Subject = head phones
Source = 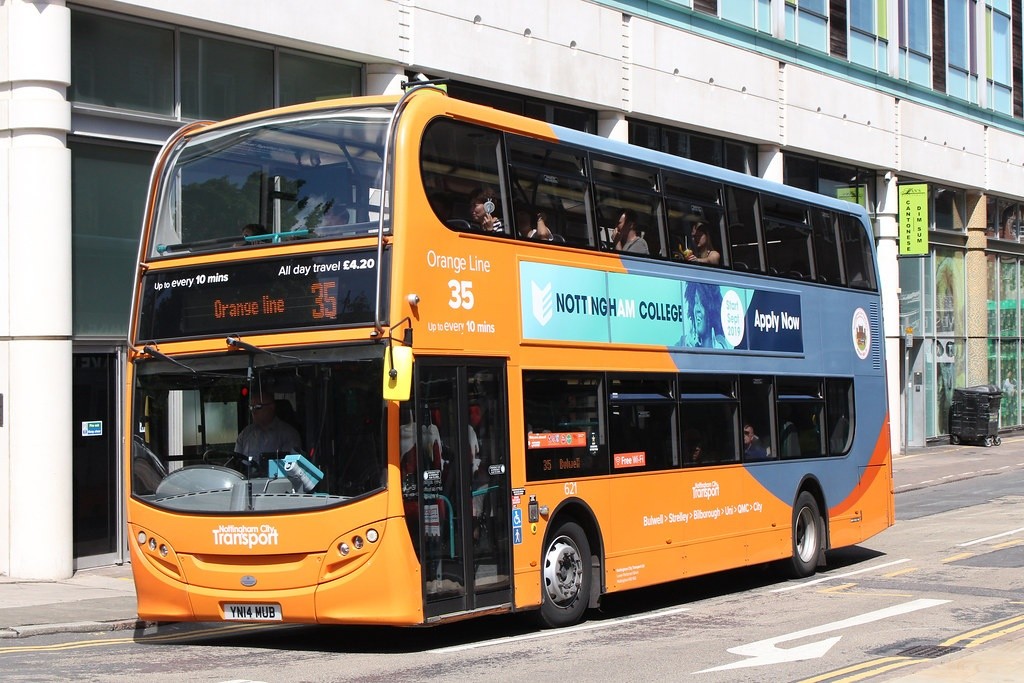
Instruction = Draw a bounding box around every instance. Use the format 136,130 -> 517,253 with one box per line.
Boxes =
483,198 -> 495,214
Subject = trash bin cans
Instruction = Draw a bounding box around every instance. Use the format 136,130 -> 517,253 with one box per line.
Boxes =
950,385 -> 1004,447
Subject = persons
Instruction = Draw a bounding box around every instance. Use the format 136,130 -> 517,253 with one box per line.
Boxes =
232,224 -> 269,247
744,425 -> 767,460
464,185 -> 503,232
611,208 -> 650,255
688,223 -> 721,265
514,201 -> 553,241
314,205 -> 350,236
675,283 -> 728,350
233,390 -> 302,479
686,441 -> 703,462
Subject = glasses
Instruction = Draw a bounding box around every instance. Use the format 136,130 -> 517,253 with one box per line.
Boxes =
248,404 -> 271,411
682,438 -> 704,445
743,431 -> 749,435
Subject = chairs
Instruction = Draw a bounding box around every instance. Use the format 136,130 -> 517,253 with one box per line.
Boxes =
779,422 -> 800,458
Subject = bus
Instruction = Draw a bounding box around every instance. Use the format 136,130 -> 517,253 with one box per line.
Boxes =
123,85 -> 896,630
